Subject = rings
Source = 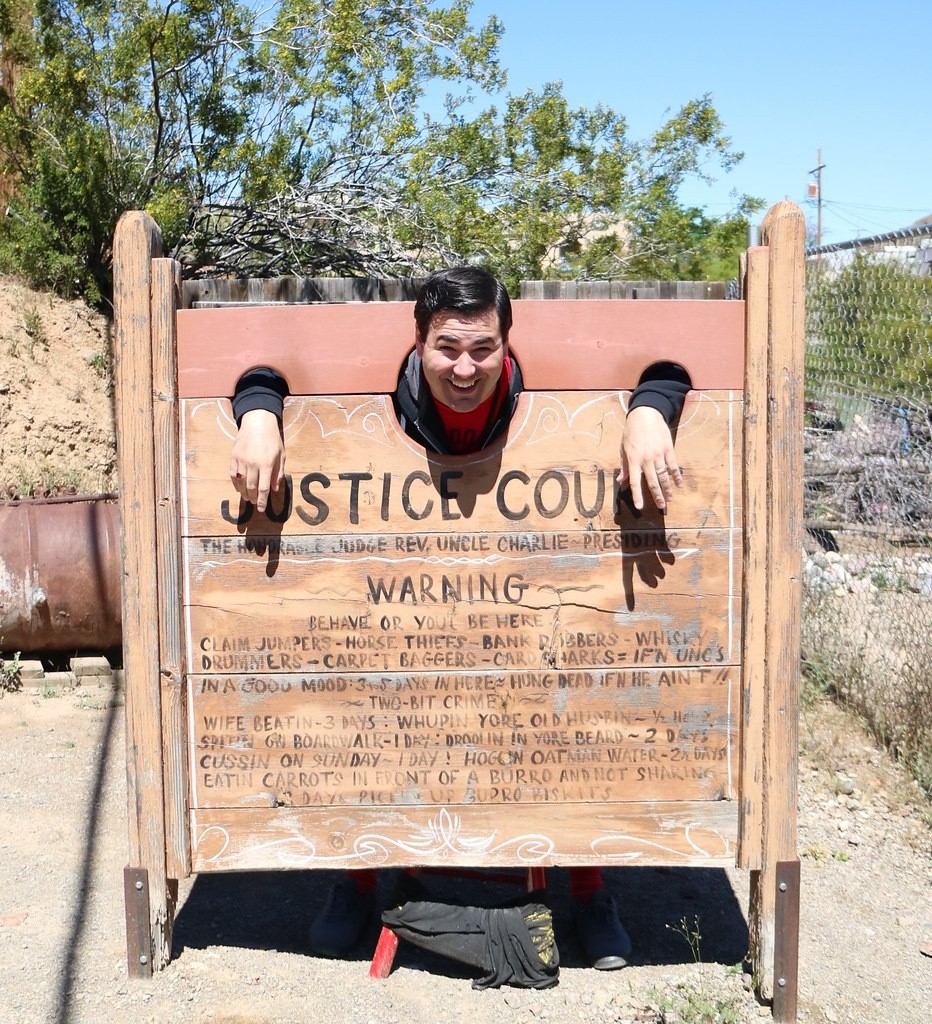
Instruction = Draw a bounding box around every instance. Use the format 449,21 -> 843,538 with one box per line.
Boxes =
657,467 -> 667,475
237,472 -> 246,480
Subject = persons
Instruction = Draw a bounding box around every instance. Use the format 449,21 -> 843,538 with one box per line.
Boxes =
229,267 -> 693,969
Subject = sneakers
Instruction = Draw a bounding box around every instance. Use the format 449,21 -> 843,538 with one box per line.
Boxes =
309,872 -> 378,957
567,891 -> 632,969
